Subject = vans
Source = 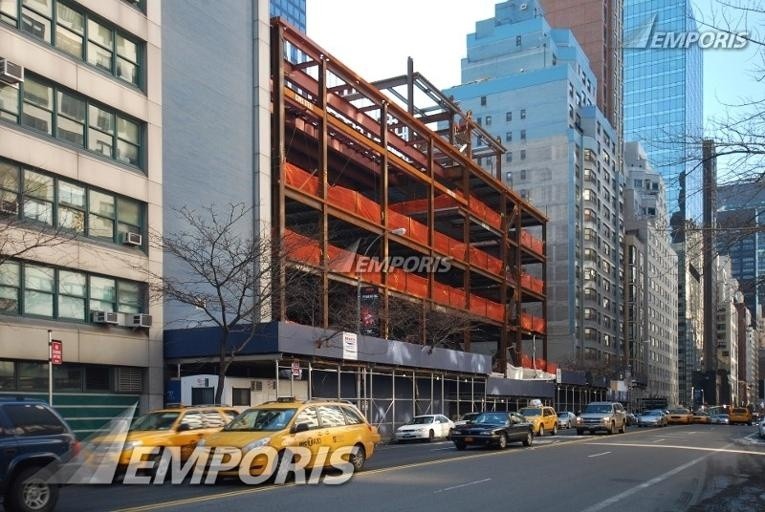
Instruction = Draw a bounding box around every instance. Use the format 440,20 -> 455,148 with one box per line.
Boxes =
520,399 -> 558,435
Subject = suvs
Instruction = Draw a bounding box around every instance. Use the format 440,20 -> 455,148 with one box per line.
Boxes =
576,402 -> 628,435
0,396 -> 81,512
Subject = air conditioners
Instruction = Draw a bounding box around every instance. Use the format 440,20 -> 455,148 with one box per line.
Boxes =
0,57 -> 26,84
92,310 -> 119,326
132,313 -> 153,328
123,230 -> 142,246
0,197 -> 20,216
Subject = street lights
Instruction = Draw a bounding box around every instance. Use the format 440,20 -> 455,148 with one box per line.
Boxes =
356,228 -> 406,414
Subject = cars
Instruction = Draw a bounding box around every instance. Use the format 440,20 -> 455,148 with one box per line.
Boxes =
624,405 -> 765,438
396,414 -> 456,443
452,411 -> 535,451
82,404 -> 248,485
556,412 -> 578,429
188,396 -> 381,484
455,412 -> 487,426
